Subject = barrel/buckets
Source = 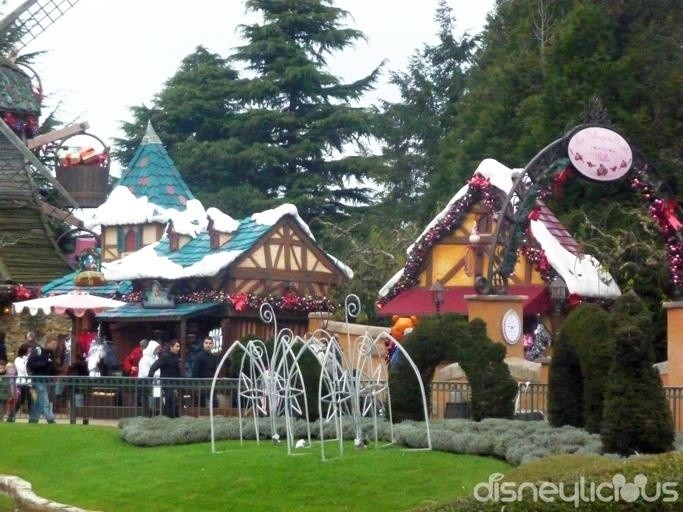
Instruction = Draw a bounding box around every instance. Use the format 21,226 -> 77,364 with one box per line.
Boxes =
55,133 -> 111,208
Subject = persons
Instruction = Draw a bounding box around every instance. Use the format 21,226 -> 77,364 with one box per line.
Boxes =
0,330 -> 222,427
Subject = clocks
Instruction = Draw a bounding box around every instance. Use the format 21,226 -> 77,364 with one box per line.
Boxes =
502,309 -> 522,345
